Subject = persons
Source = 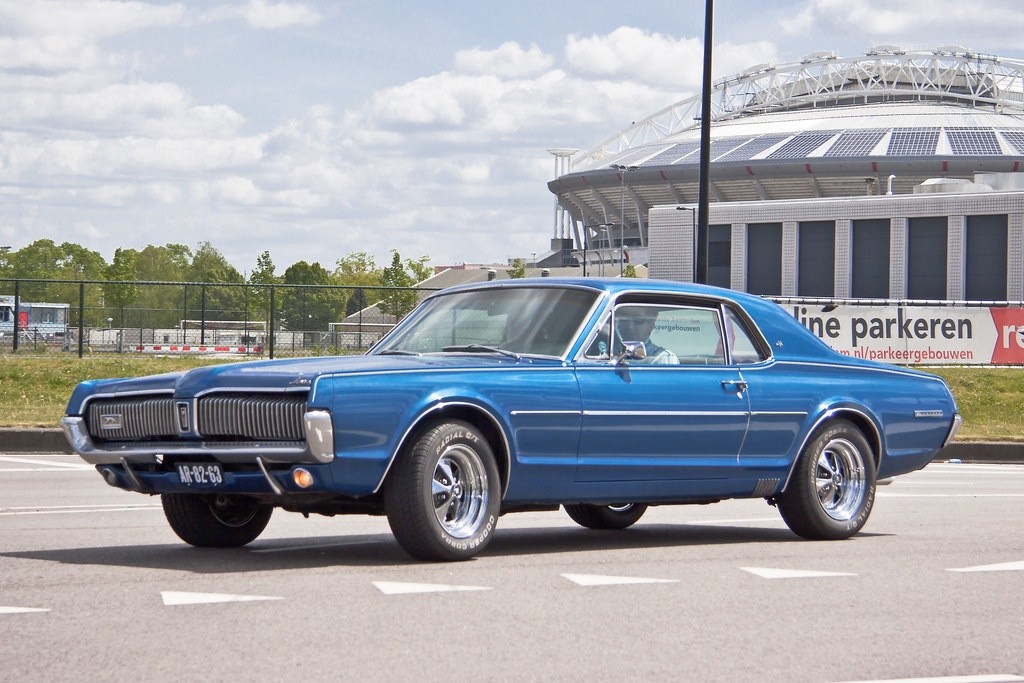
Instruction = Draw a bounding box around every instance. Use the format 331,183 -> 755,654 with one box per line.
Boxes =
619,306 -> 680,364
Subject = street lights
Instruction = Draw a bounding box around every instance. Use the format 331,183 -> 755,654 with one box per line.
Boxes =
583,222 -> 613,273
676,206 -> 697,280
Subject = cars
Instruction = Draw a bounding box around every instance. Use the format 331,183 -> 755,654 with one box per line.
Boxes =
58,276 -> 965,562
